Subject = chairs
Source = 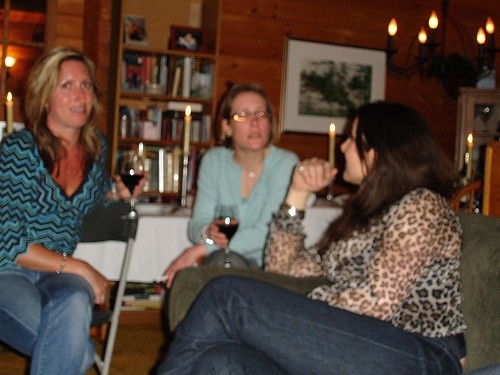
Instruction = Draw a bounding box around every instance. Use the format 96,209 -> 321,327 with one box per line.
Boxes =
77,202 -> 138,375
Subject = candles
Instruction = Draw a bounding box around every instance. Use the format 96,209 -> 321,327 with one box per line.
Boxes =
183,105 -> 192,154
6,92 -> 14,134
466,133 -> 474,178
328,122 -> 336,168
387,12 -> 496,75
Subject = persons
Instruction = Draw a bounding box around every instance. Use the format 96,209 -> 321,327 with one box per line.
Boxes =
154,101 -> 462,375
164,83 -> 298,288
124,18 -> 143,41
0,49 -> 148,375
179,34 -> 198,50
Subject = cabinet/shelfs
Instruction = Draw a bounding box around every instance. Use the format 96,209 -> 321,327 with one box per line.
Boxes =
456,87 -> 500,215
109,0 -> 221,208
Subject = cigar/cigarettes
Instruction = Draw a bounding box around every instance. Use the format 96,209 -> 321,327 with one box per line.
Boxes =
156,274 -> 168,283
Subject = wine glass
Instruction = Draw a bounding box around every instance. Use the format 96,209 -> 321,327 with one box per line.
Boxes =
121,152 -> 145,220
214,202 -> 239,271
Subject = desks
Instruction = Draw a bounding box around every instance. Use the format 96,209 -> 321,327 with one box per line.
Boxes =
72,202 -> 342,284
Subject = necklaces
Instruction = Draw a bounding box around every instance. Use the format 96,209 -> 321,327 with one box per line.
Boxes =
239,154 -> 266,178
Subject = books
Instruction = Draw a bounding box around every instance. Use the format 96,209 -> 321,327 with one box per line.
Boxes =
117,53 -> 213,194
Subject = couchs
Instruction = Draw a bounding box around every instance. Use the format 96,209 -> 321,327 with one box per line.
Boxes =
170,209 -> 500,375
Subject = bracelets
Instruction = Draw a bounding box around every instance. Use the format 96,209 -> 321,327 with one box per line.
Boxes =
111,183 -> 118,203
205,226 -> 214,246
56,251 -> 67,274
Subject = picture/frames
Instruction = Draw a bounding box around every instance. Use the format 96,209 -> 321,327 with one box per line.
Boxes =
124,13 -> 148,48
170,24 -> 209,54
277,33 -> 389,136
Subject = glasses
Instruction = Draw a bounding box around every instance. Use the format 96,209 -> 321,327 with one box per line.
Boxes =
227,109 -> 269,122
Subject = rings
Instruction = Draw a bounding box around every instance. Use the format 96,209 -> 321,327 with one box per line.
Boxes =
298,165 -> 306,174
100,292 -> 106,300
